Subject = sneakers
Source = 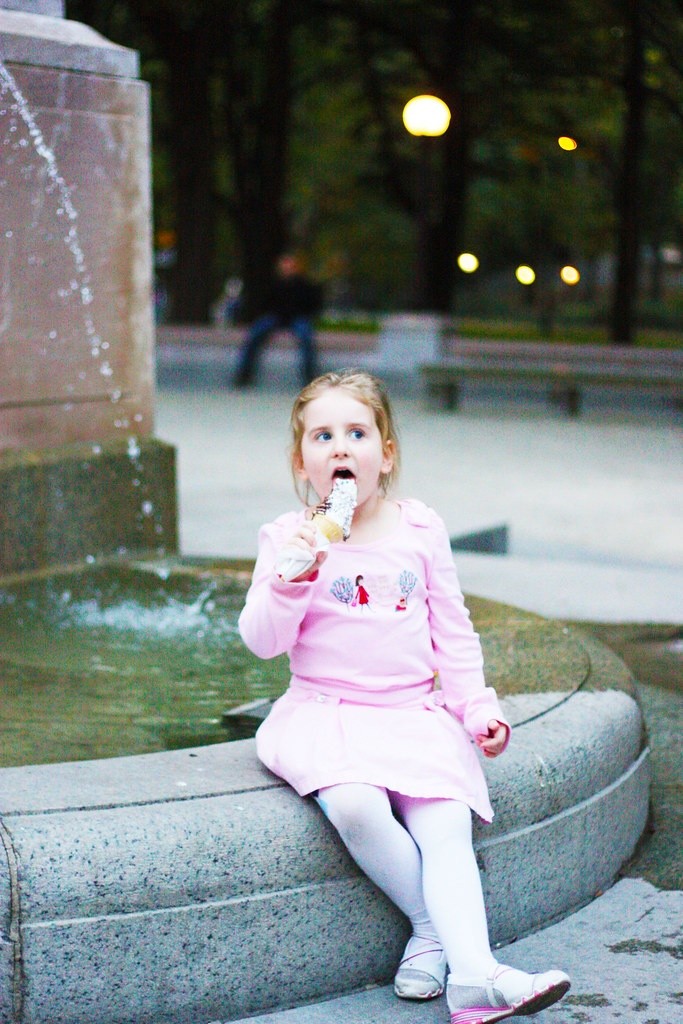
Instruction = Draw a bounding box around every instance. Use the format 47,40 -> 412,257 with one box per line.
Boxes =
394,932 -> 448,999
446,961 -> 573,1024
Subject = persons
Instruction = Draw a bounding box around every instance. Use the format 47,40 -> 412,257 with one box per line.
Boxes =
233,249 -> 324,389
237,369 -> 571,1023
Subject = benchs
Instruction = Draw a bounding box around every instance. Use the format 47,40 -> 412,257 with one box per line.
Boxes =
425,338 -> 683,417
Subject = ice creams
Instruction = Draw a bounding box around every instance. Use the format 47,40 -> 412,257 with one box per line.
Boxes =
310,477 -> 357,543
